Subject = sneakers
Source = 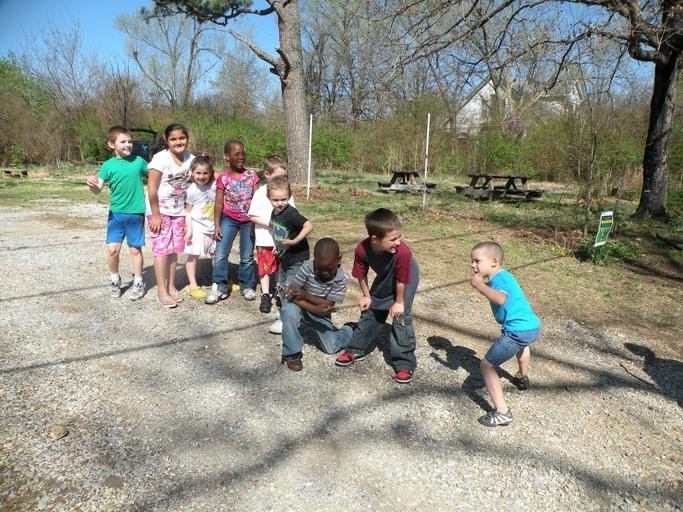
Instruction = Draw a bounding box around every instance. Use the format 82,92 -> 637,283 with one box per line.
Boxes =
344,321 -> 358,329
110,276 -> 122,297
283,354 -> 302,371
259,293 -> 271,313
395,368 -> 413,383
240,288 -> 256,300
512,371 -> 529,390
269,311 -> 283,333
479,406 -> 514,428
335,350 -> 365,366
129,281 -> 148,300
205,290 -> 227,304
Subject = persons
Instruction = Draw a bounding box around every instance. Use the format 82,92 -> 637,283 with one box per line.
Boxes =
206,140 -> 260,307
277,237 -> 358,372
86,126 -> 149,302
184,156 -> 240,298
144,123 -> 220,308
336,208 -> 422,385
470,242 -> 542,427
248,154 -> 296,313
266,177 -> 314,336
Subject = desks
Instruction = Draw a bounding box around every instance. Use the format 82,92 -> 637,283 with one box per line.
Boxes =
467,174 -> 532,191
390,169 -> 420,185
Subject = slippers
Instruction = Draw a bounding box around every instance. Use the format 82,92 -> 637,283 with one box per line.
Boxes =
185,286 -> 207,298
228,281 -> 240,292
168,290 -> 184,302
155,294 -> 176,308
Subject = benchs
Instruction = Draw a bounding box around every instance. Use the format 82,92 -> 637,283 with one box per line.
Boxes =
1,168 -> 27,179
